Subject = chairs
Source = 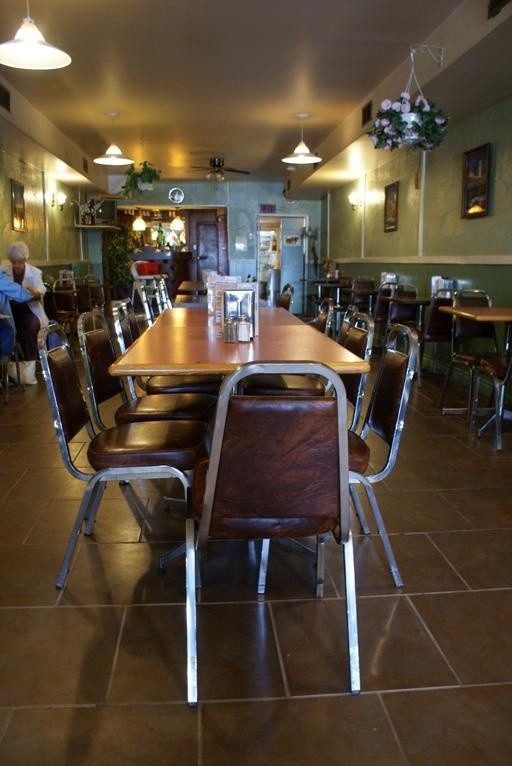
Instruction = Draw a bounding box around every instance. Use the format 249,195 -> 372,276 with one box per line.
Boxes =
334,275 -> 352,312
317,298 -> 333,336
159,278 -> 172,309
373,282 -> 398,323
257,324 -> 418,593
471,356 -> 512,450
388,284 -> 417,332
153,279 -> 163,314
438,288 -> 499,429
122,299 -> 141,341
326,313 -> 375,431
351,275 -> 374,305
44,282 -> 58,322
0,352 -> 20,404
418,289 -> 456,388
77,311 -> 217,520
136,285 -> 155,326
52,279 -> 78,311
160,361 -> 362,708
37,324 -> 209,591
107,300 -> 241,401
244,305 -> 358,396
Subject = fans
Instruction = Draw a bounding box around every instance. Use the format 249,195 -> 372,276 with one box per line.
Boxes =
190,156 -> 251,176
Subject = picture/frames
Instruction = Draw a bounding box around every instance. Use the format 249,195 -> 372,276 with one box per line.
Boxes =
384,181 -> 398,232
11,180 -> 26,232
461,143 -> 490,218
59,271 -> 73,288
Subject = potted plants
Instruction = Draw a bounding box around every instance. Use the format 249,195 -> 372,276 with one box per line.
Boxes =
80,203 -> 97,225
127,162 -> 162,193
119,185 -> 136,200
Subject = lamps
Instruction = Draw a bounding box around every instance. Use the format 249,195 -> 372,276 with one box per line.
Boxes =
0,17 -> 72,70
93,144 -> 135,166
281,141 -> 322,165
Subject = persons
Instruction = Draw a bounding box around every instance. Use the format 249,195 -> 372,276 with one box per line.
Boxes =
3,242 -> 48,361
1,267 -> 33,396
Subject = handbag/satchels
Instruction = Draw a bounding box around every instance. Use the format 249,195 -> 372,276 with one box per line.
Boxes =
8,342 -> 37,385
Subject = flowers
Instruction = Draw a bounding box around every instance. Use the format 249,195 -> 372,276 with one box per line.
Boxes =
366,91 -> 452,149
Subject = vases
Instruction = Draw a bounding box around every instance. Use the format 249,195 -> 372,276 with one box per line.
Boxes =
400,114 -> 421,140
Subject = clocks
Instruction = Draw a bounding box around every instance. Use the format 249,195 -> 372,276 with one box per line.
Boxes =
168,187 -> 185,203
